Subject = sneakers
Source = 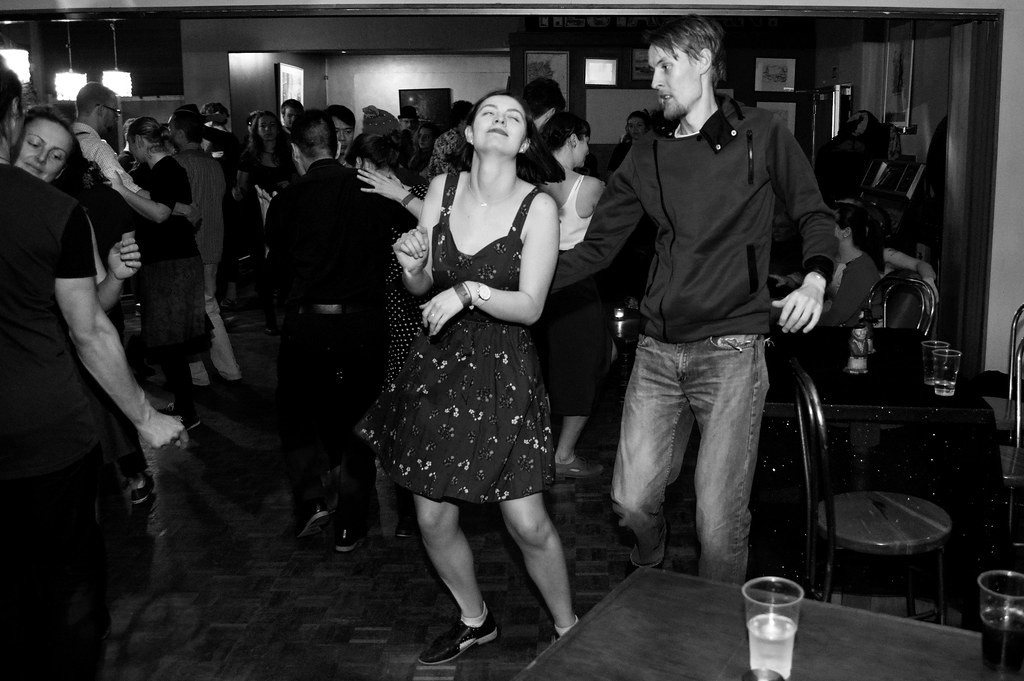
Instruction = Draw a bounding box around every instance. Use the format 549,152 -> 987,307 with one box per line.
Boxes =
334,521 -> 367,552
418,610 -> 497,665
297,502 -> 328,538
554,455 -> 603,481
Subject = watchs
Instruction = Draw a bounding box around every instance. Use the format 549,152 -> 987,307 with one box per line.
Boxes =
471,281 -> 491,307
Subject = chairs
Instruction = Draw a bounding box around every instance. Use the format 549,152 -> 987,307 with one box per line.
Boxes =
789,270 -> 1024,627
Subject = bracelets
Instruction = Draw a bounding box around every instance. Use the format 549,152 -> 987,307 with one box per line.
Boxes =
400,193 -> 417,208
453,282 -> 472,308
463,281 -> 474,300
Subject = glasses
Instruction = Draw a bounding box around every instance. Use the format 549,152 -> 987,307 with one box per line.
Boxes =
95,104 -> 121,117
260,123 -> 278,128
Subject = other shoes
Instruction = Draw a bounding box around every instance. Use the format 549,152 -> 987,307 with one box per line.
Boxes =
182,414 -> 201,432
397,521 -> 415,537
159,402 -> 190,416
129,473 -> 153,505
221,380 -> 265,404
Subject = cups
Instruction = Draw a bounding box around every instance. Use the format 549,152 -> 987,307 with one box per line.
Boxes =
932,349 -> 962,396
741,668 -> 785,681
741,576 -> 805,679
977,570 -> 1024,672
921,340 -> 950,386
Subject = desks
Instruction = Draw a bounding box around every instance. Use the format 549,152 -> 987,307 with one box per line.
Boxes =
509,565 -> 986,681
752,328 -> 1016,608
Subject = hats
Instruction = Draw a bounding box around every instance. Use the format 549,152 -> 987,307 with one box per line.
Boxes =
398,106 -> 419,118
363,106 -> 399,137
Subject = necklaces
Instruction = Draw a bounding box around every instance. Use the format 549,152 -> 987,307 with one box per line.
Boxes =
466,178 -> 518,207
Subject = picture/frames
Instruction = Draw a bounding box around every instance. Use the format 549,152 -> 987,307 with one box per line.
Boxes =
523,50 -> 569,112
753,56 -> 797,95
630,48 -> 654,82
755,99 -> 798,139
882,18 -> 915,127
582,56 -> 619,87
278,63 -> 305,126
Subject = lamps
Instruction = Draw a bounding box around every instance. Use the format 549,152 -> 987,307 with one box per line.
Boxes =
51,18 -> 88,102
99,18 -> 132,98
0,20 -> 31,83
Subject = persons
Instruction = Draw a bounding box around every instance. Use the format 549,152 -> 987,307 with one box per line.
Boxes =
356,89 -> 580,665
546,14 -> 840,586
11,77 -> 937,587
0,62 -> 191,681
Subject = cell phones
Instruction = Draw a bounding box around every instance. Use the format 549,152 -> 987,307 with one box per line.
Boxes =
181,416 -> 201,430
